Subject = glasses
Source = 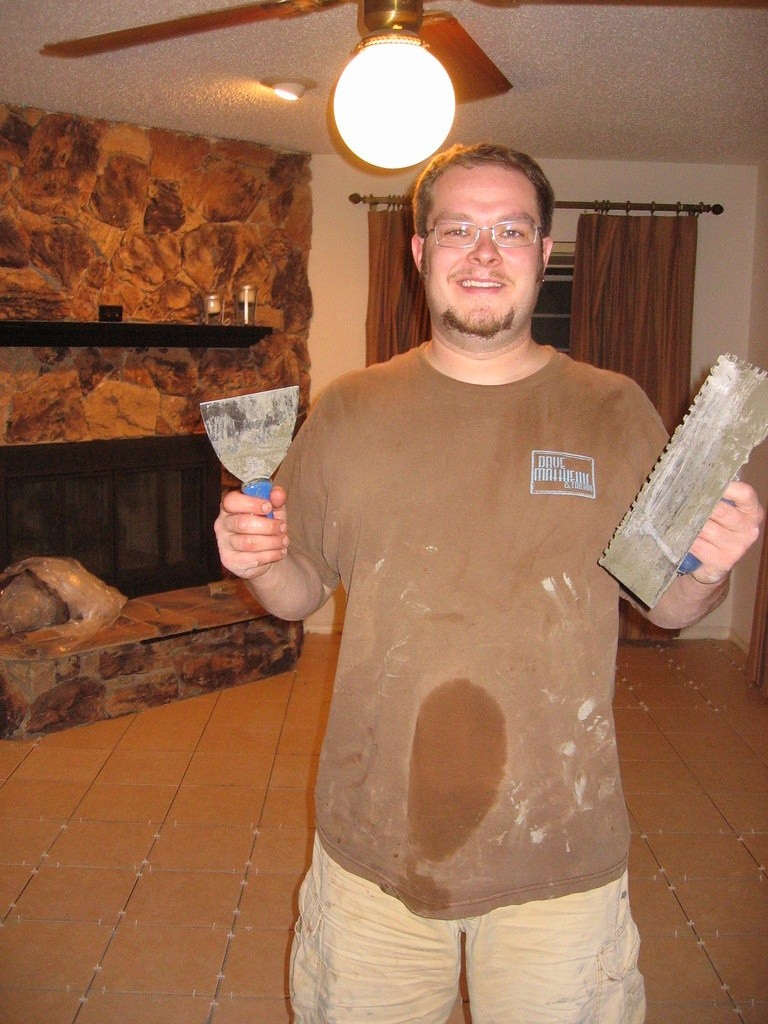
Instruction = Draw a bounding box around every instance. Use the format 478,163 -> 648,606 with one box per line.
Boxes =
424,218 -> 544,249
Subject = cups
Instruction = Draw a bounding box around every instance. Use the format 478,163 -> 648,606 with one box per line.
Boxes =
236,285 -> 257,326
204,296 -> 224,326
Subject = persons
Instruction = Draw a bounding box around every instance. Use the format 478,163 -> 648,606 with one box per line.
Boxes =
213,142 -> 765,1024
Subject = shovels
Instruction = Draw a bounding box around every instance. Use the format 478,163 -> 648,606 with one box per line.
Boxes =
196,384 -> 301,523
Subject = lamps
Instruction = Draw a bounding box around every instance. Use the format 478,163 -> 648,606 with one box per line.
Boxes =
331,0 -> 455,173
262,77 -> 317,101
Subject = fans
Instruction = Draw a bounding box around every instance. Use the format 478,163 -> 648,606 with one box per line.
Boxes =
39,0 -> 768,103
